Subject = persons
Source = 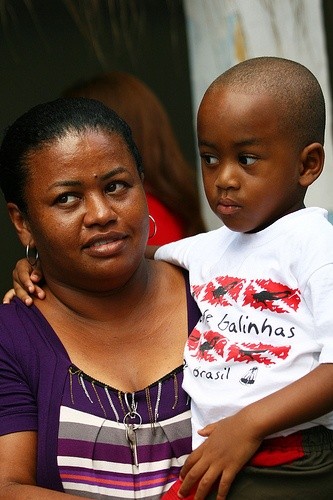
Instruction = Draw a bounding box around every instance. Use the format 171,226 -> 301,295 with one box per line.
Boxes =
0,94 -> 202,500
58,68 -> 206,246
2,56 -> 333,500
